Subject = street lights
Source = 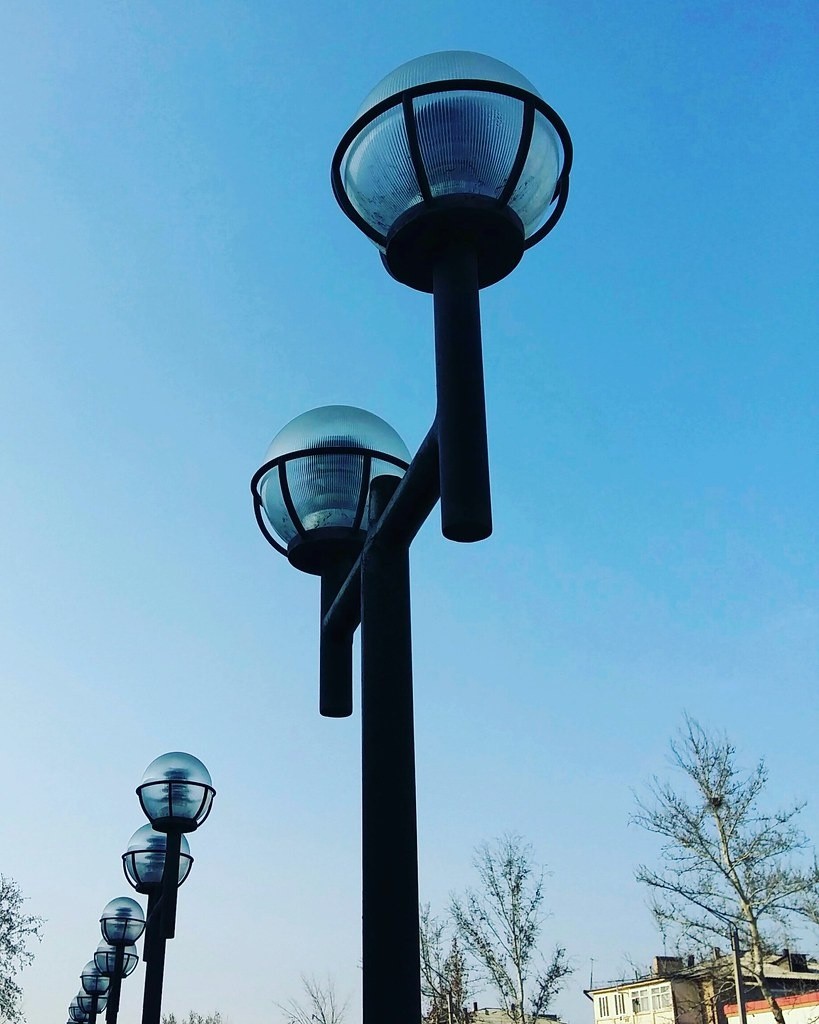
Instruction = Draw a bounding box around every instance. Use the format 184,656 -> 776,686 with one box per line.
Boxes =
67,895 -> 146,1024
252,49 -> 576,1024
121,750 -> 217,1024
